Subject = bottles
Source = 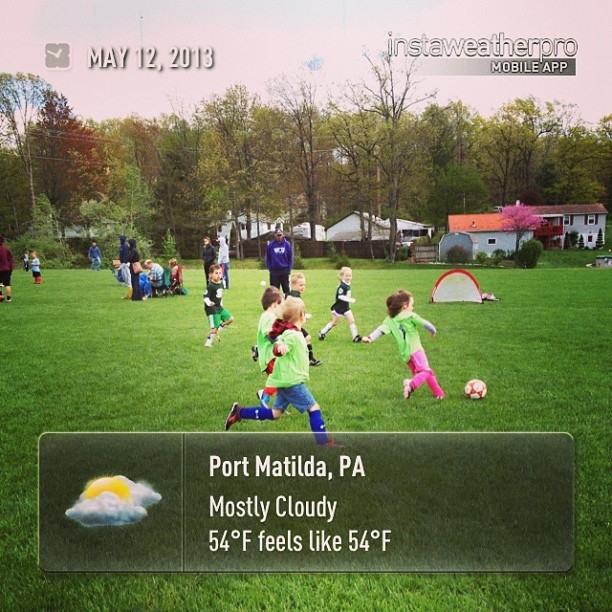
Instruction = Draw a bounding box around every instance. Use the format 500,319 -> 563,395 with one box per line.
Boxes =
275,229 -> 283,236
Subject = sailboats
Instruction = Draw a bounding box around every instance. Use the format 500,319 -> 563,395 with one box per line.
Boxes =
306,314 -> 313,320
260,281 -> 267,287
465,378 -> 487,400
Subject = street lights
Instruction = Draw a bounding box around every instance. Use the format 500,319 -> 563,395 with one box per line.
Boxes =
131,261 -> 144,273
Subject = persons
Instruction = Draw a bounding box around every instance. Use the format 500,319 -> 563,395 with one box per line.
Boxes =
116,236 -> 128,281
265,229 -> 293,293
201,236 -> 215,287
362,289 -> 446,398
203,265 -> 234,348
318,266 -> 362,342
17,250 -> 28,272
149,259 -> 163,296
0,236 -> 14,304
88,242 -> 102,271
28,252 -> 42,285
285,272 -> 323,366
215,237 -> 230,289
168,258 -> 178,292
127,239 -> 141,301
224,295 -> 346,451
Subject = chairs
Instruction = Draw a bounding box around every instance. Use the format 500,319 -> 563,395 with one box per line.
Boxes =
322,439 -> 346,451
354,336 -> 361,342
206,339 -> 214,348
404,378 -> 413,401
319,332 -> 325,340
310,360 -> 321,365
252,346 -> 257,360
224,403 -> 241,431
437,392 -> 446,398
